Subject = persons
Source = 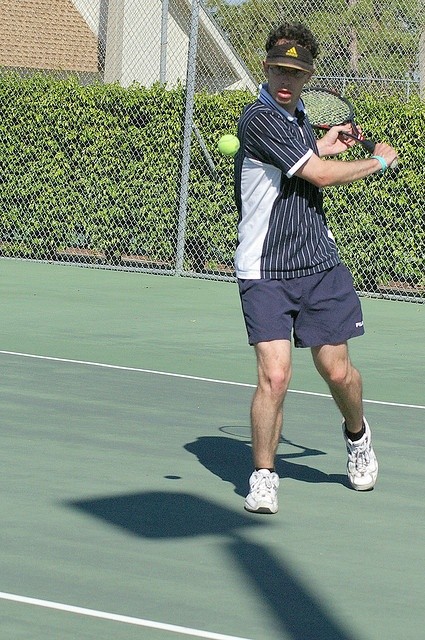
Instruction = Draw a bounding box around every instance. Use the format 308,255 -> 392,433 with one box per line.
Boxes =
233,21 -> 379,513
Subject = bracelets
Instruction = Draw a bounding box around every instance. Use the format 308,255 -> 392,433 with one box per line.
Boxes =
370,155 -> 387,174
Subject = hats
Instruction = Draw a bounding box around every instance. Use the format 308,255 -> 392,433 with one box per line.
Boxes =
262,44 -> 314,74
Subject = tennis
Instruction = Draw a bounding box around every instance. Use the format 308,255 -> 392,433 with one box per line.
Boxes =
218,134 -> 240,156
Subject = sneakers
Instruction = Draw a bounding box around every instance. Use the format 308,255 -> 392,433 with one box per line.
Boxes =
243,467 -> 279,513
340,415 -> 378,491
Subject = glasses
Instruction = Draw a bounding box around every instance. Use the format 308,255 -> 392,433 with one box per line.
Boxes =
266,64 -> 306,80
